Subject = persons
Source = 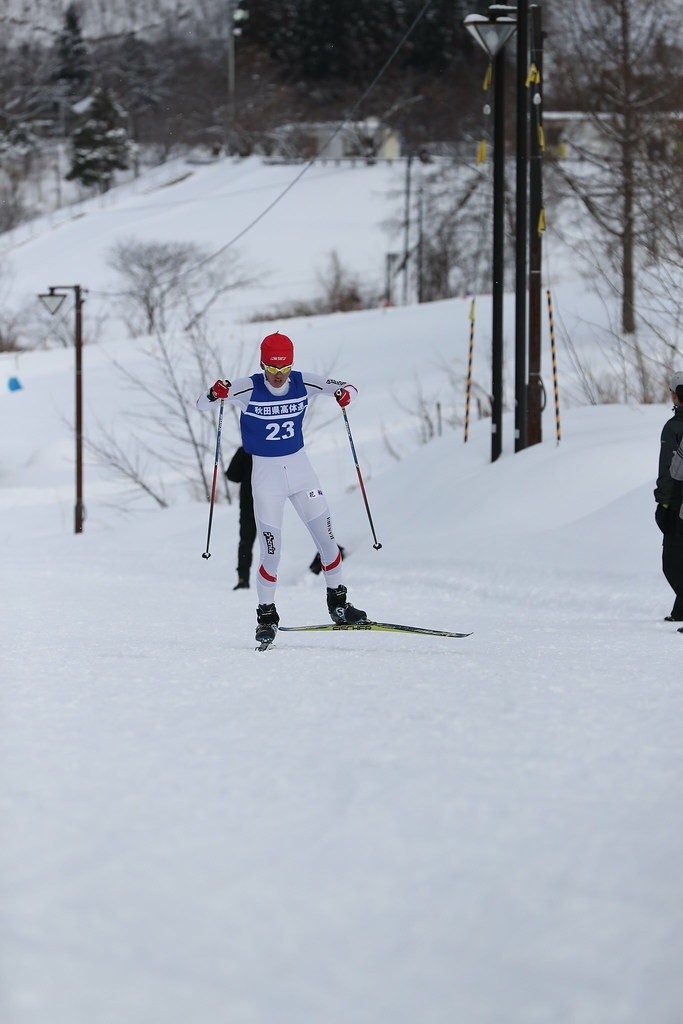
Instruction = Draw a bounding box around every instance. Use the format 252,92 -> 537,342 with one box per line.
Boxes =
197,331 -> 367,643
654,371 -> 683,632
225,446 -> 257,590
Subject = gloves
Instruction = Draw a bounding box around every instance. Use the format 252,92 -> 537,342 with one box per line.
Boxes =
207,379 -> 232,402
334,388 -> 350,407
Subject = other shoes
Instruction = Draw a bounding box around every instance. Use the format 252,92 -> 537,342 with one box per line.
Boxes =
665,616 -> 683,622
233,581 -> 249,590
677,628 -> 683,633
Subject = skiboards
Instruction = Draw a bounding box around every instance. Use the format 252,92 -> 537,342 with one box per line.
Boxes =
255,617 -> 474,656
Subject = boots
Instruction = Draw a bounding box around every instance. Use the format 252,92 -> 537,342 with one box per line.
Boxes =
255,604 -> 280,642
327,585 -> 370,625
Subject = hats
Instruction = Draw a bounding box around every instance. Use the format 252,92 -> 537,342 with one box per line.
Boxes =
668,371 -> 683,393
260,331 -> 293,365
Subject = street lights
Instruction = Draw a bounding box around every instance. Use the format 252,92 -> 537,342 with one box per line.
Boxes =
462,12 -> 517,467
34,283 -> 90,541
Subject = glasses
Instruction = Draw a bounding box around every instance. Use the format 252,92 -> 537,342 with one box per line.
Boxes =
265,366 -> 291,375
